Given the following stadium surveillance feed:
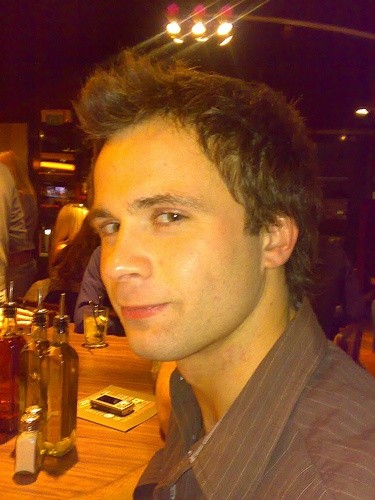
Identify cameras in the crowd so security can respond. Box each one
[91,394,135,416]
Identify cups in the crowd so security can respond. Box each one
[83,306,108,346]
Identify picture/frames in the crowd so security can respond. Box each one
[38,107,73,148]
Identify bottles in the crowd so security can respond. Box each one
[40,314,79,458]
[14,406,45,478]
[0,301,27,434]
[17,307,53,420]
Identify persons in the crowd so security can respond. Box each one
[41,210,101,321]
[0,162,37,308]
[74,245,125,336]
[41,203,89,312]
[70,48,375,499]
[0,150,37,284]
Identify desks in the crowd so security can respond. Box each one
[0,323,166,500]
